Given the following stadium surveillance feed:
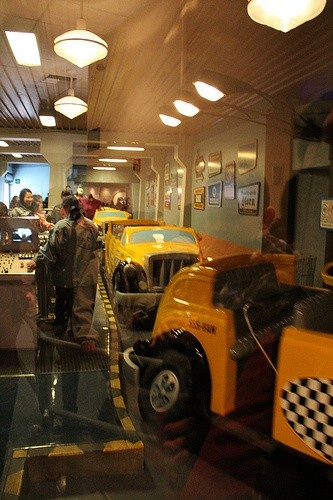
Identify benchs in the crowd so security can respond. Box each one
[210,262,333,335]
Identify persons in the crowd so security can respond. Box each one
[260,207,301,257]
[0,185,127,231]
[26,195,99,353]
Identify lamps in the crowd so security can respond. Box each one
[158,70,325,142]
[54,70,89,119]
[245,0,326,34]
[54,0,109,69]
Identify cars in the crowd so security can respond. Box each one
[92,207,332,476]
